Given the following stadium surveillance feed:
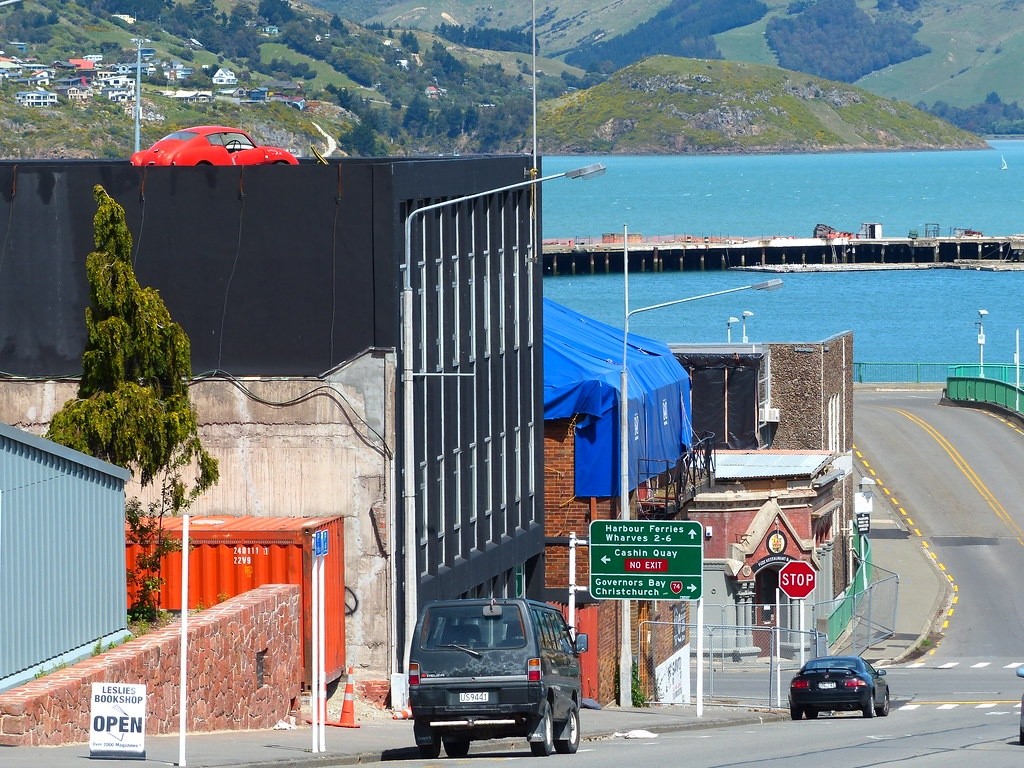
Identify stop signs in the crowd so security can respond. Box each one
[779,560,817,599]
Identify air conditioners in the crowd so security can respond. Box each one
[765,409,781,424]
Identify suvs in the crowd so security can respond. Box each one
[407,598,590,761]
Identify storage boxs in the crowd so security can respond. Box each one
[126,515,347,685]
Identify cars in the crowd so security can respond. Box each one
[789,654,890,720]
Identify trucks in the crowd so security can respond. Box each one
[121,516,346,704]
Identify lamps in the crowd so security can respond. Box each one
[727,317,739,341]
[742,311,753,343]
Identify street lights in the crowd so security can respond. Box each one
[403,163,608,677]
[129,37,152,152]
[619,222,783,706]
[977,310,990,378]
[727,315,740,342]
[741,311,754,342]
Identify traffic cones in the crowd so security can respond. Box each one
[304,672,337,726]
[393,705,414,720]
[331,668,363,729]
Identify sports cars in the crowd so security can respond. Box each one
[131,126,300,167]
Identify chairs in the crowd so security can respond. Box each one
[497,621,526,647]
[446,626,485,647]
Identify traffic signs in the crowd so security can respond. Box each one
[587,518,704,602]
